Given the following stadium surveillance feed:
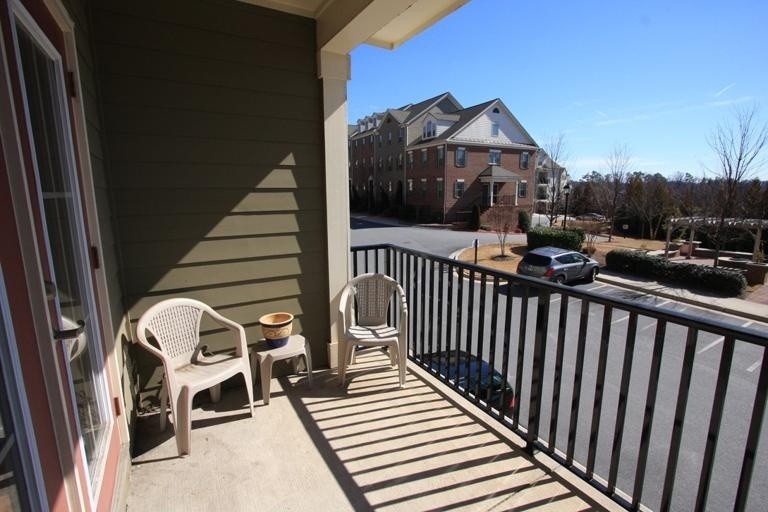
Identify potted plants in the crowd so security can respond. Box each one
[746,240,768,286]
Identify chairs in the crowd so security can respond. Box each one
[60,316,88,362]
[337,273,408,389]
[136,298,254,458]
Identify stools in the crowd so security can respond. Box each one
[250,334,312,405]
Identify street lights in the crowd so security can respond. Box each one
[564,183,570,230]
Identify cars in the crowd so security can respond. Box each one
[576,213,605,222]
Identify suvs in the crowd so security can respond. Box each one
[413,350,513,419]
[517,247,599,293]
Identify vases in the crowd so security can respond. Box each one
[258,312,294,347]
